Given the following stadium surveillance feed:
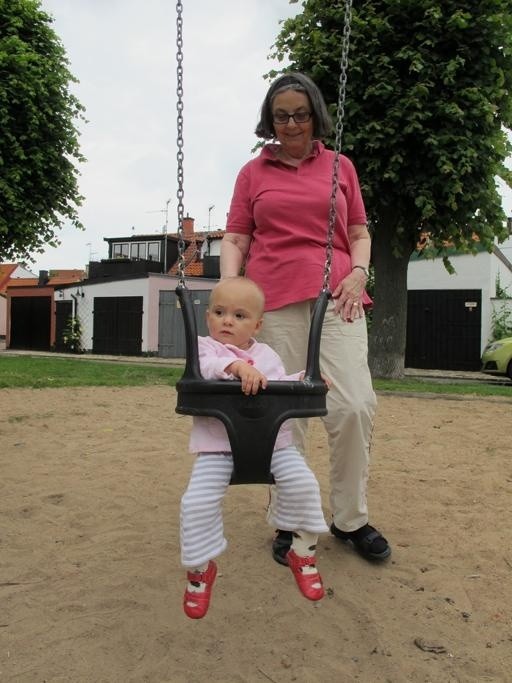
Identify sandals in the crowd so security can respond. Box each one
[329,520,392,560]
[273,528,293,566]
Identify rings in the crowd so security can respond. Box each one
[353,301,358,306]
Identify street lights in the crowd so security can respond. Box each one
[166,199,173,234]
[208,205,217,231]
[85,243,92,261]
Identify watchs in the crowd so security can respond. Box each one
[350,265,370,278]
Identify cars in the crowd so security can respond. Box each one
[481,336,511,380]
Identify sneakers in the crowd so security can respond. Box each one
[184,559,217,619]
[287,550,325,600]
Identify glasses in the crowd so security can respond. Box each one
[271,112,311,123]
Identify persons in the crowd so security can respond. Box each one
[220,72,389,570]
[177,277,328,620]
